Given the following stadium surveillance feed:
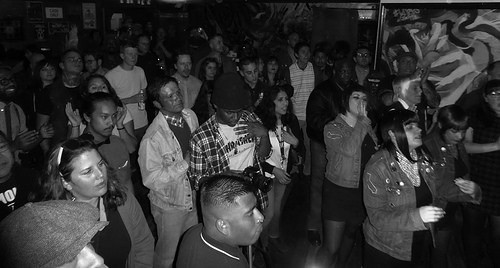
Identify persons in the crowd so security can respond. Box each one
[0,8,500,268]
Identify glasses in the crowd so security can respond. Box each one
[159,88,181,103]
[0,77,16,86]
[356,52,369,57]
[85,59,97,65]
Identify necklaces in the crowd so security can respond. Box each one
[216,122,239,156]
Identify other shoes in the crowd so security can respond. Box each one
[308,230,321,247]
[248,250,266,268]
[269,239,293,254]
[263,249,273,266]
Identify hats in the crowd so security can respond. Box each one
[264,84,294,98]
[211,73,252,111]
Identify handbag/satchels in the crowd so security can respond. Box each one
[18,166,39,197]
[289,130,307,178]
